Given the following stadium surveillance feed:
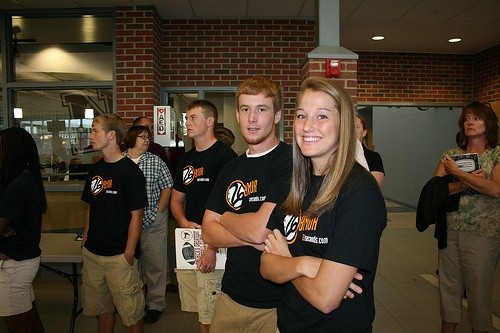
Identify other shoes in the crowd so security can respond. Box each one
[165,283,179,293]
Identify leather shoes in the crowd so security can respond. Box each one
[144,305,161,324]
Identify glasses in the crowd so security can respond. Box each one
[137,135,150,140]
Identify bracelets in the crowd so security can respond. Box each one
[459,182,463,189]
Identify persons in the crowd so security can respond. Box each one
[435,101,500,333]
[200,76,293,333]
[259,76,387,333]
[0,126,49,333]
[80,100,237,333]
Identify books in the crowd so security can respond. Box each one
[449,152,479,195]
[174,226,227,270]
